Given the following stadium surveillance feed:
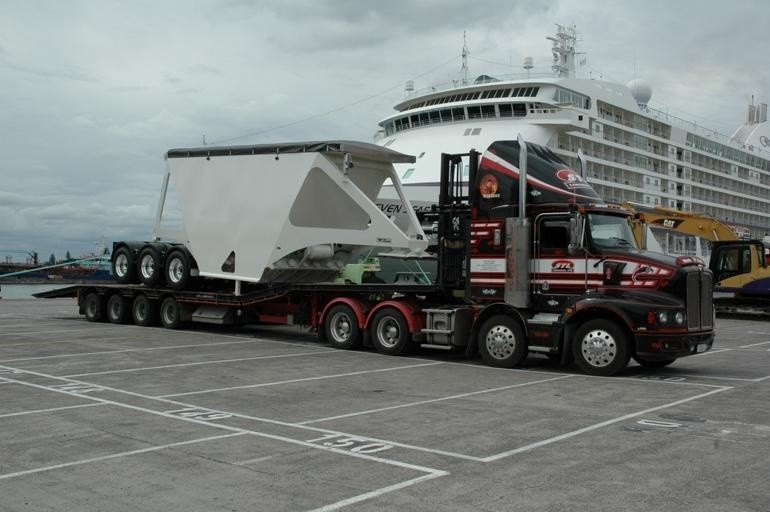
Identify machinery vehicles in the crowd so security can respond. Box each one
[614,201,769,312]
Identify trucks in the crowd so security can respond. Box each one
[33,136,714,377]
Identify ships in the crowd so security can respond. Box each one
[376,22,769,233]
